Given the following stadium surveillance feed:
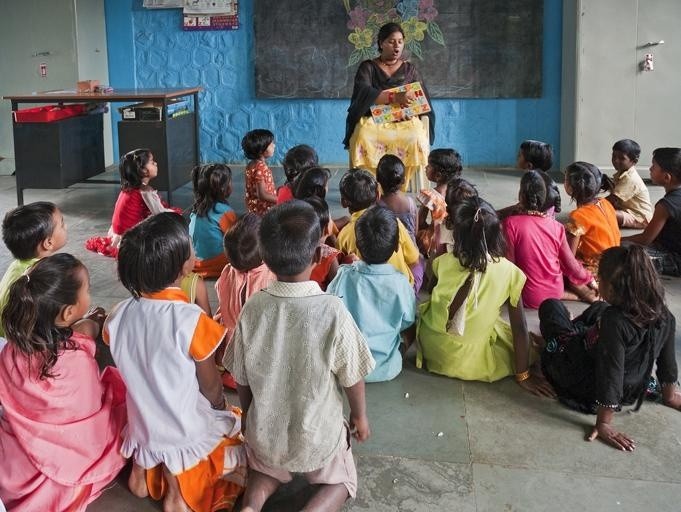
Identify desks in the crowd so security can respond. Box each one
[2,86,203,207]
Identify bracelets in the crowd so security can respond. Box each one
[513,370,531,385]
[388,92,394,104]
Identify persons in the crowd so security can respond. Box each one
[497,140,562,216]
[423,149,462,256]
[103,213,248,510]
[563,161,621,285]
[210,212,274,388]
[302,198,343,290]
[336,166,423,294]
[275,145,350,229]
[292,168,339,247]
[603,138,653,229]
[1,200,107,338]
[85,147,182,256]
[376,155,418,251]
[326,206,419,384]
[242,128,281,214]
[221,200,375,512]
[499,170,597,307]
[187,163,241,277]
[424,180,478,290]
[178,273,210,315]
[415,196,558,399]
[621,148,680,277]
[530,243,680,452]
[343,22,434,192]
[3,255,125,511]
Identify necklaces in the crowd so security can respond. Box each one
[377,55,401,67]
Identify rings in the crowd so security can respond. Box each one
[407,100,410,102]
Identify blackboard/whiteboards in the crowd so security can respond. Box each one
[251,0,544,101]
